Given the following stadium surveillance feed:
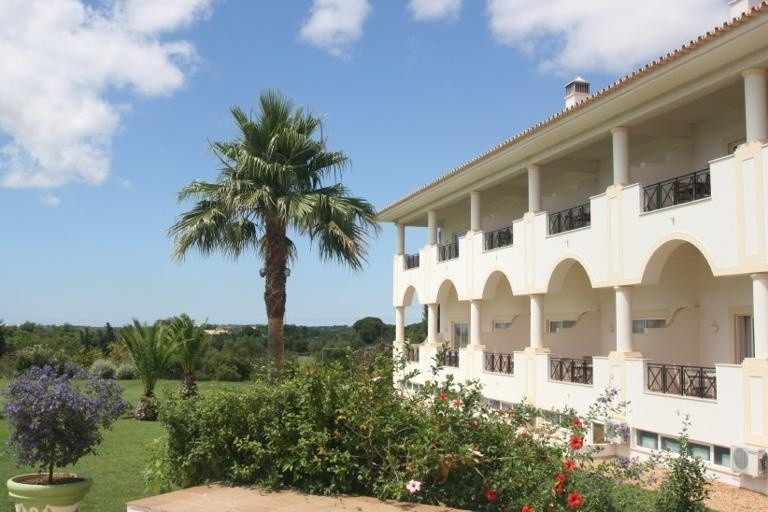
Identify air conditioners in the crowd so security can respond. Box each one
[730,444,767,478]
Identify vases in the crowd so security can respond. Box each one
[6,473,92,512]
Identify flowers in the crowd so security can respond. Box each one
[0,358,127,485]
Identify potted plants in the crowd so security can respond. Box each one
[121,318,195,420]
[162,313,217,401]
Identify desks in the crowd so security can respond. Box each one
[690,372,716,400]
[567,364,592,385]
[675,182,704,204]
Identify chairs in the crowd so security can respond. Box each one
[674,362,696,395]
